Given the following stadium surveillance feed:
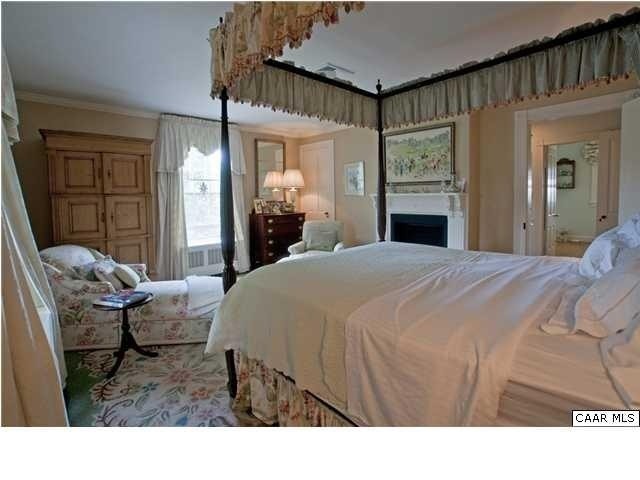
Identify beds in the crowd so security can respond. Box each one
[205,239,640,427]
[39,245,225,351]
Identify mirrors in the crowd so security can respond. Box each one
[254,138,286,204]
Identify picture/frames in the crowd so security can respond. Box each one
[556,157,575,188]
[381,122,455,185]
[253,200,264,214]
[344,161,365,197]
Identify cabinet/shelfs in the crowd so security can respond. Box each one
[38,129,157,281]
[249,212,306,271]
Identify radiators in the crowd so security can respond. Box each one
[181,241,238,276]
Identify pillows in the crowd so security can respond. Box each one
[305,230,337,251]
[579,213,640,280]
[539,245,640,338]
[93,261,125,291]
[114,263,140,288]
[72,253,116,282]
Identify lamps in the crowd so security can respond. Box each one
[263,171,284,201]
[280,169,305,213]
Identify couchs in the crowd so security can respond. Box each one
[288,220,345,260]
